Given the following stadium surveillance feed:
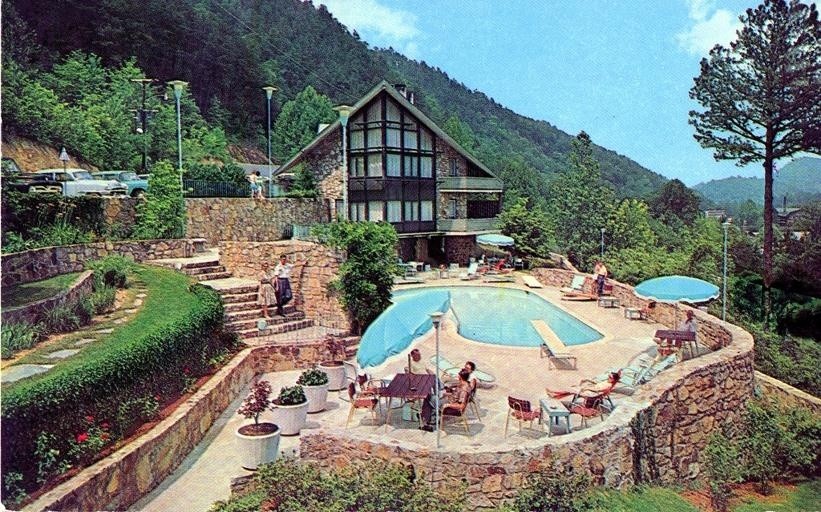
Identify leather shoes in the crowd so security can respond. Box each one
[416,412,424,420]
[420,426,431,431]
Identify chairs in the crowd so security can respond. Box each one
[624,301,658,325]
[346,373,388,427]
[559,273,587,293]
[560,368,622,431]
[439,377,482,432]
[578,343,689,396]
[504,396,544,441]
[390,255,543,289]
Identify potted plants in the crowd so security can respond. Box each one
[270,384,309,436]
[315,332,348,391]
[235,376,281,470]
[294,368,330,413]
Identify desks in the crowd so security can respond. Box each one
[538,398,571,438]
[597,296,621,308]
[381,373,436,437]
[655,329,699,358]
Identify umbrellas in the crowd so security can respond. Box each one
[633,276,721,329]
[477,234,514,263]
[356,290,453,369]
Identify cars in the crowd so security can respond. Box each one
[1,156,154,200]
[130,173,154,180]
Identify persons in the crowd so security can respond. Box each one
[544,373,618,406]
[481,251,512,271]
[255,171,265,199]
[417,369,470,432]
[274,255,308,315]
[675,310,697,349]
[592,259,608,295]
[405,348,443,394]
[250,171,258,198]
[465,361,477,399]
[259,262,277,319]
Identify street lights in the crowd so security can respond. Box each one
[165,78,190,193]
[329,101,355,222]
[719,222,732,321]
[260,86,278,197]
[599,228,606,256]
[129,76,168,174]
[426,310,447,447]
